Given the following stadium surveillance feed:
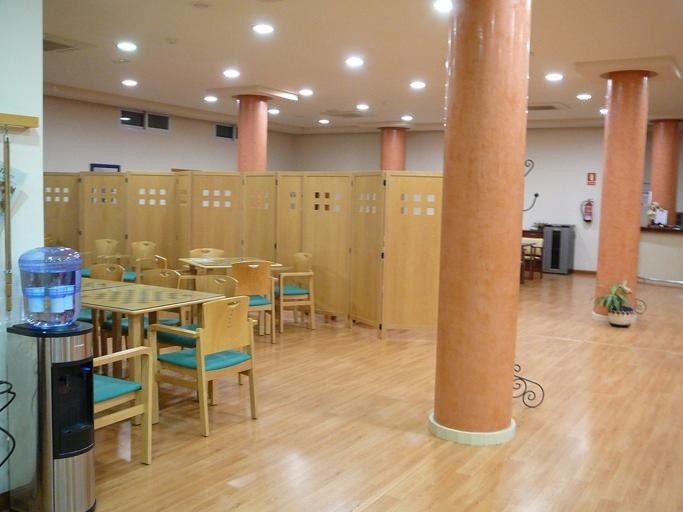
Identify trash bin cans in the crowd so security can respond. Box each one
[544,225,575,274]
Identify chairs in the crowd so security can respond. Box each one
[80,267,239,425]
[79,238,316,346]
[520,230,544,282]
[148,295,260,437]
[79,343,154,465]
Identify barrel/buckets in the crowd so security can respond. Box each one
[19,245,83,333]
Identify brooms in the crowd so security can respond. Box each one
[1,112,40,313]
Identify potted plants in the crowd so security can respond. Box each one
[592,281,636,327]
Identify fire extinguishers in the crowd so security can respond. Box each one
[581,199,594,221]
